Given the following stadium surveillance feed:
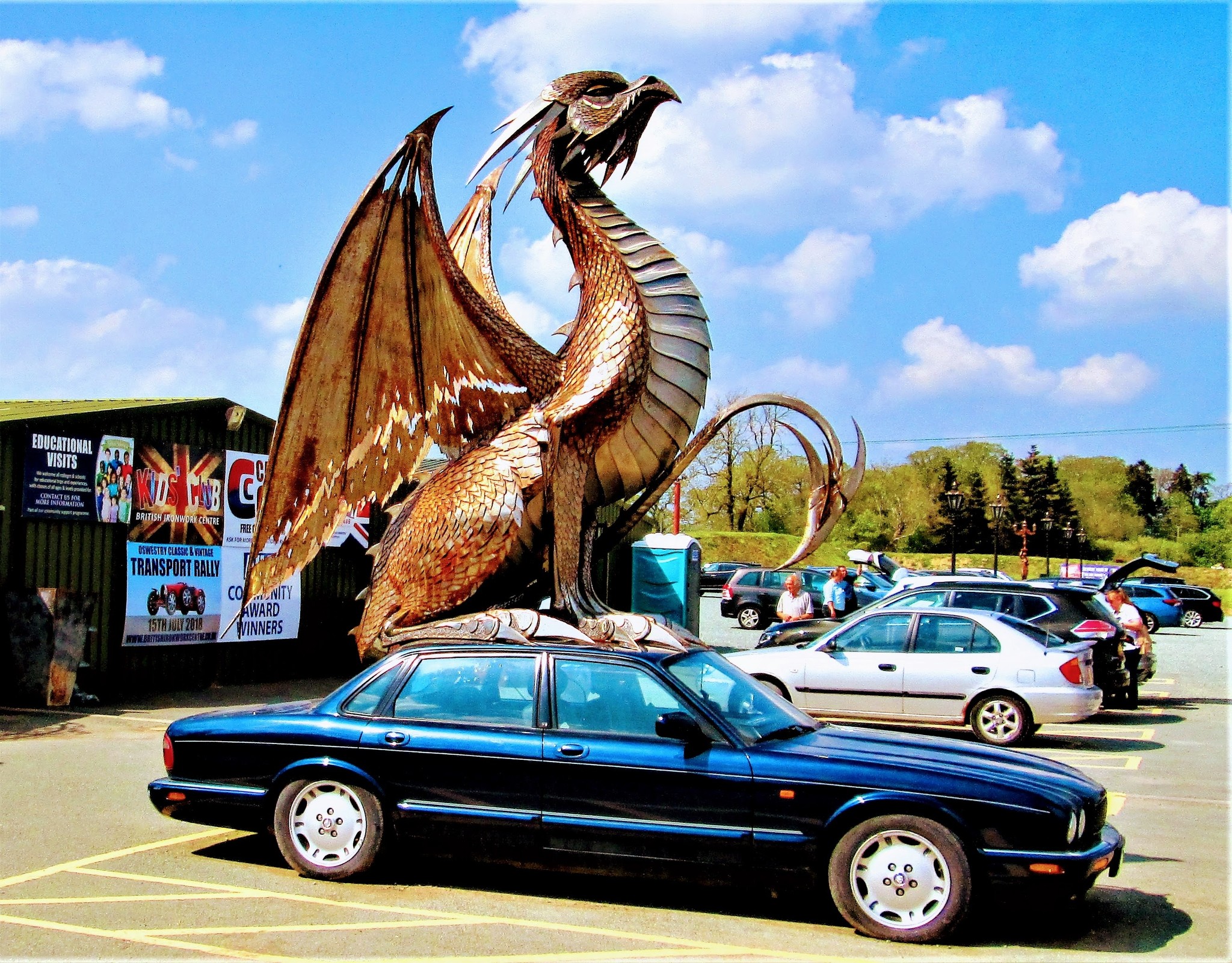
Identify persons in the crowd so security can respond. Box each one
[95,449,132,523]
[1107,590,1152,710]
[822,564,862,618]
[776,574,814,622]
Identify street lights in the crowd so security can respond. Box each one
[1039,511,1055,578]
[1062,522,1074,577]
[988,493,1008,576]
[1013,519,1037,580]
[944,480,964,574]
[1076,527,1088,577]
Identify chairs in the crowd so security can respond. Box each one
[524,668,571,727]
[593,668,633,732]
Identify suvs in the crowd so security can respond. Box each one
[699,562,762,597]
[1023,551,1224,635]
[752,550,1180,705]
[799,548,1014,607]
[721,567,833,631]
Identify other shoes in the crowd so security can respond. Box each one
[1125,693,1138,710]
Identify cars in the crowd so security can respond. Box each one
[146,582,205,616]
[698,607,1104,747]
[148,642,1126,946]
